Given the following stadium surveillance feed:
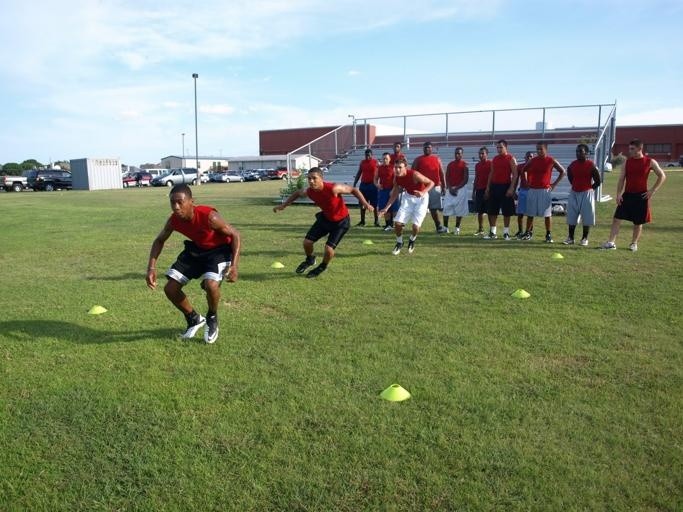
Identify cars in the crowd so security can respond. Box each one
[121,167,302,187]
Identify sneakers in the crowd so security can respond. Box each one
[182,314,206,338]
[374,221,381,227]
[382,224,394,231]
[562,236,574,245]
[391,241,404,256]
[474,231,485,236]
[512,231,533,240]
[356,222,366,226]
[503,232,511,240]
[205,315,219,343]
[306,263,327,278]
[454,227,460,236]
[296,256,316,273]
[601,241,616,250]
[580,237,589,245]
[629,243,638,251]
[436,224,449,233]
[545,233,554,243]
[483,229,498,239]
[407,238,415,253]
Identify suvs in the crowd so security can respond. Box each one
[27,170,72,191]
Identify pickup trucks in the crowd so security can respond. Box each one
[0,170,32,193]
[665,156,682,167]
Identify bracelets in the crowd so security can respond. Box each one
[147,267,156,270]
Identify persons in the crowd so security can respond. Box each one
[597,140,666,252]
[562,144,601,245]
[352,140,566,255]
[273,167,375,278]
[135,172,142,188]
[146,183,241,344]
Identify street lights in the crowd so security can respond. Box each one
[191,72,201,175]
[181,133,186,159]
